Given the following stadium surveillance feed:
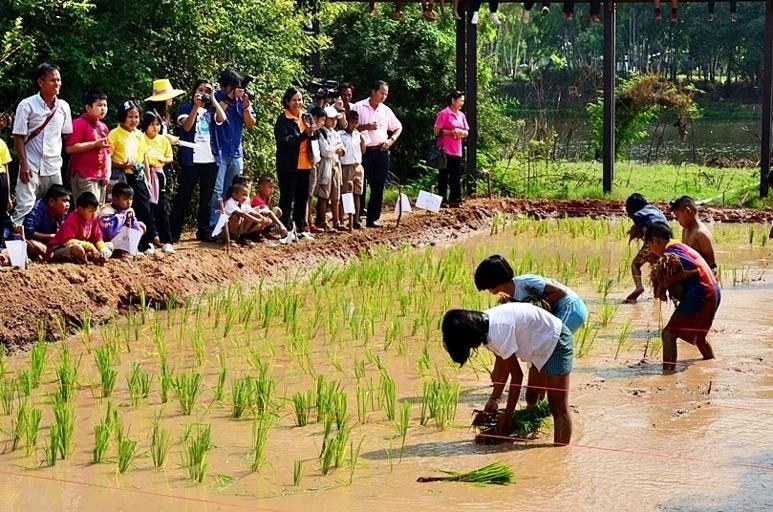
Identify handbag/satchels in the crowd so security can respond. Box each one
[425,144,448,169]
[307,130,322,165]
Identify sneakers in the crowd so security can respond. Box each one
[137,242,156,256]
[304,224,325,234]
[160,243,176,254]
[230,230,315,250]
[322,225,338,234]
[333,225,350,231]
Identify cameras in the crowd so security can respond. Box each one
[134,163,143,171]
[198,94,211,104]
[241,74,256,100]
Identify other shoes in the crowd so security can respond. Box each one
[395,8,404,17]
[452,11,461,20]
[590,10,600,23]
[565,12,573,21]
[424,10,435,20]
[654,12,661,20]
[707,15,713,22]
[489,13,501,25]
[369,7,375,16]
[523,10,530,24]
[541,7,549,16]
[471,14,479,24]
[728,16,736,21]
[441,7,448,18]
[670,14,678,21]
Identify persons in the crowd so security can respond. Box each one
[442,301,576,449]
[274,80,403,245]
[645,222,721,374]
[669,196,719,279]
[212,173,288,250]
[473,255,587,413]
[620,193,669,304]
[432,89,470,209]
[1,61,256,265]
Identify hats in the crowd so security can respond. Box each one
[323,106,342,119]
[143,78,187,103]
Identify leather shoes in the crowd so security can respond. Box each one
[448,200,464,208]
[366,219,383,228]
[346,216,363,227]
[440,202,450,208]
